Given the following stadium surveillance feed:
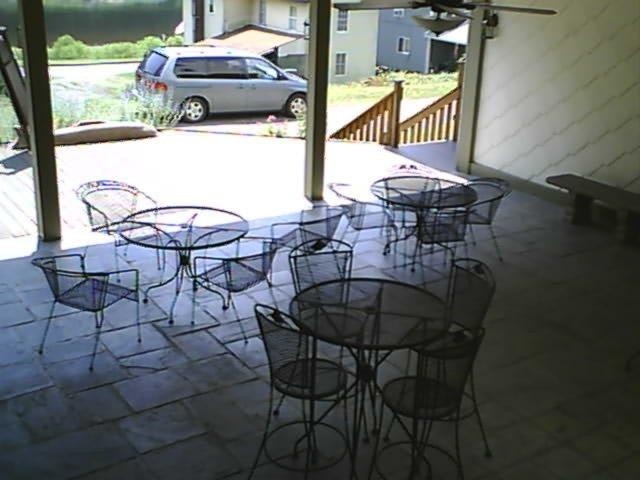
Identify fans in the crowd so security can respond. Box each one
[333,1,557,23]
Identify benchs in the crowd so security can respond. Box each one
[545,173,640,245]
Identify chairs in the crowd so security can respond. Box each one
[31,171,512,480]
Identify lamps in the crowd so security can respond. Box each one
[409,7,466,38]
[483,12,500,39]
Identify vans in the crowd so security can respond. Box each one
[132,41,319,135]
